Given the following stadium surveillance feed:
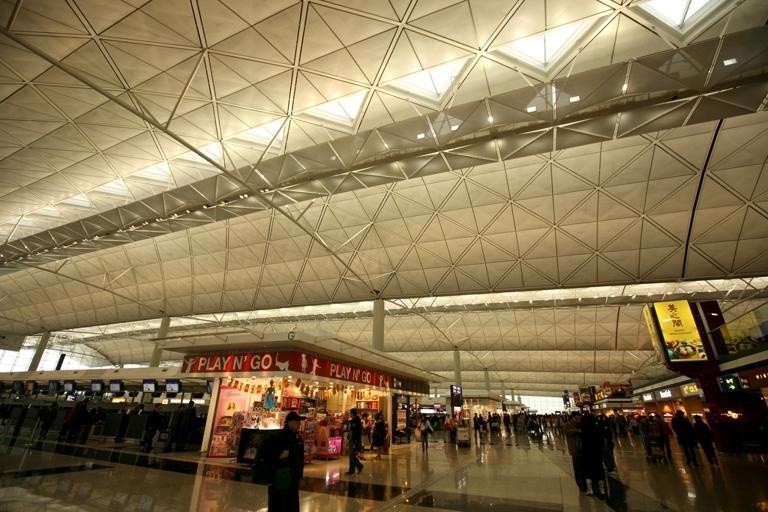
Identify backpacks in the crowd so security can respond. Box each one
[249,432,291,486]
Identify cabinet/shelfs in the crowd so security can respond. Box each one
[357,400,383,421]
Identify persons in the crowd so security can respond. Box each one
[28,398,196,454]
[472,409,719,466]
[263,411,306,512]
[566,412,608,499]
[343,408,386,474]
[417,414,440,451]
[263,379,275,411]
[445,414,457,444]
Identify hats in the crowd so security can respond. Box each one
[284,411,308,422]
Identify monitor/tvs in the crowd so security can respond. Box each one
[48,380,61,394]
[109,379,124,393]
[143,379,158,393]
[25,380,37,391]
[165,379,182,393]
[64,380,76,392]
[91,379,105,392]
[207,381,213,394]
